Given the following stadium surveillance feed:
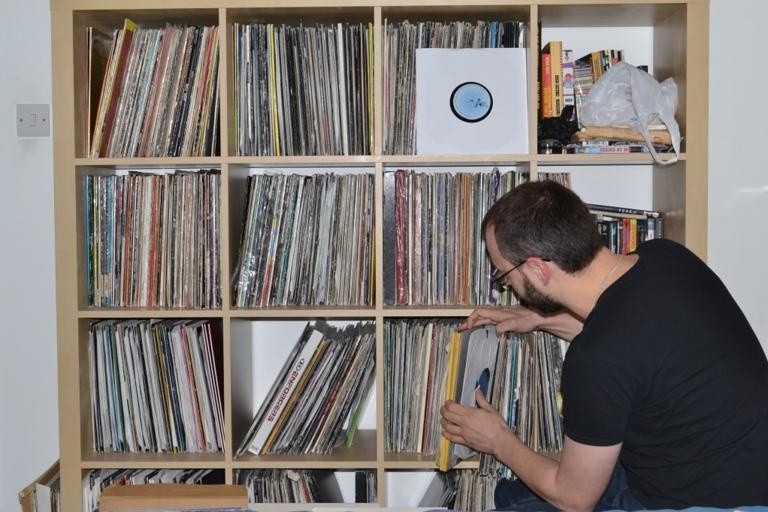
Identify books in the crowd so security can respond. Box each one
[537,172,571,191]
[233,319,375,458]
[440,468,524,512]
[81,468,225,511]
[75,15,222,158]
[383,169,533,307]
[235,172,373,310]
[583,200,666,256]
[16,461,58,511]
[86,323,223,453]
[232,20,372,157]
[385,15,521,155]
[383,315,567,483]
[237,468,376,503]
[84,171,220,311]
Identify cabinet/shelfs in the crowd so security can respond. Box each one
[50,1,707,512]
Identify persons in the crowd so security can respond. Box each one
[538,22,681,153]
[441,182,768,512]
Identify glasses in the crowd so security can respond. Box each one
[486,253,557,294]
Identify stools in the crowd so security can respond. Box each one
[101,484,249,512]
